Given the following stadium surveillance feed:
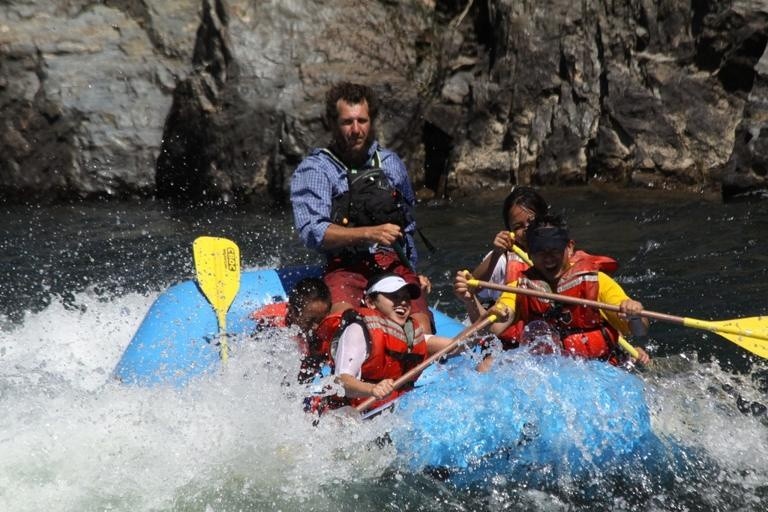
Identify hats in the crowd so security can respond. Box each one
[365,272,421,300]
[524,226,569,254]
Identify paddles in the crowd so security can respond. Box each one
[467,279,767,359]
[191,236,240,362]
[319,303,505,428]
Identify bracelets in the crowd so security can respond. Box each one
[630,318,640,320]
[464,300,474,305]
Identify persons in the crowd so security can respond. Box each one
[290,80,437,336]
[453,216,649,369]
[307,270,509,427]
[472,186,650,376]
[249,277,344,386]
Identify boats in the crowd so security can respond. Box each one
[110,267,697,496]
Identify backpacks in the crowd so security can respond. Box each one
[320,146,437,273]
